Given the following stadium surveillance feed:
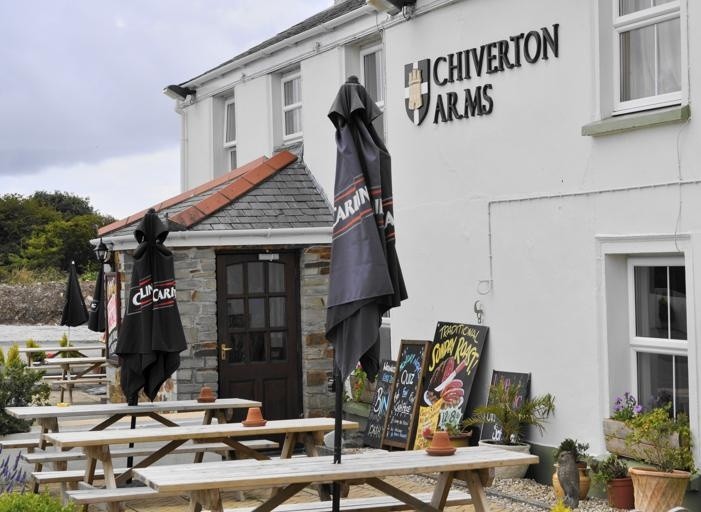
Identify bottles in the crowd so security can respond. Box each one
[431,432,449,449]
[246,408,263,421]
[201,388,212,397]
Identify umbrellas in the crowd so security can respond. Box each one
[115,208,187,481]
[88,259,115,332]
[324,76,410,512]
[59,260,90,403]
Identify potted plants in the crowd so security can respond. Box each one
[459,376,556,480]
[553,391,700,512]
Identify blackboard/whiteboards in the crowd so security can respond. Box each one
[103,272,122,367]
[380,340,433,449]
[364,358,398,449]
[480,369,531,441]
[411,321,490,450]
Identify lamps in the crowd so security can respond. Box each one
[163,83,197,102]
[92,235,111,262]
[366,0,419,18]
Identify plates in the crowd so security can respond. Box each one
[199,397,216,402]
[242,421,266,426]
[426,448,455,455]
